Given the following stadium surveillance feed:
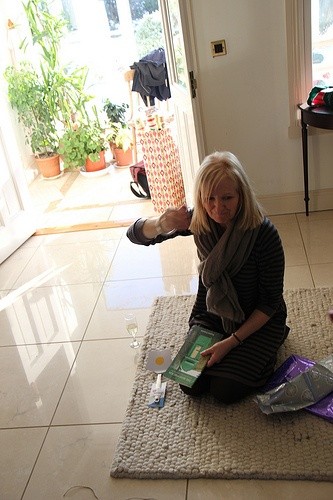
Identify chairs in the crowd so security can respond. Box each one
[124,49,169,162]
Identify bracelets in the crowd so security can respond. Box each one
[232,333,243,346]
[154,213,177,236]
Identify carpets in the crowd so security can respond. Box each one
[110,287,333,482]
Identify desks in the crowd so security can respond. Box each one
[297,103,333,217]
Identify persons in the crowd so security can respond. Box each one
[126,152,290,408]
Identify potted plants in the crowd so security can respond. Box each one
[3,0,134,178]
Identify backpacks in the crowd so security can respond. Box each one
[129,159,151,199]
